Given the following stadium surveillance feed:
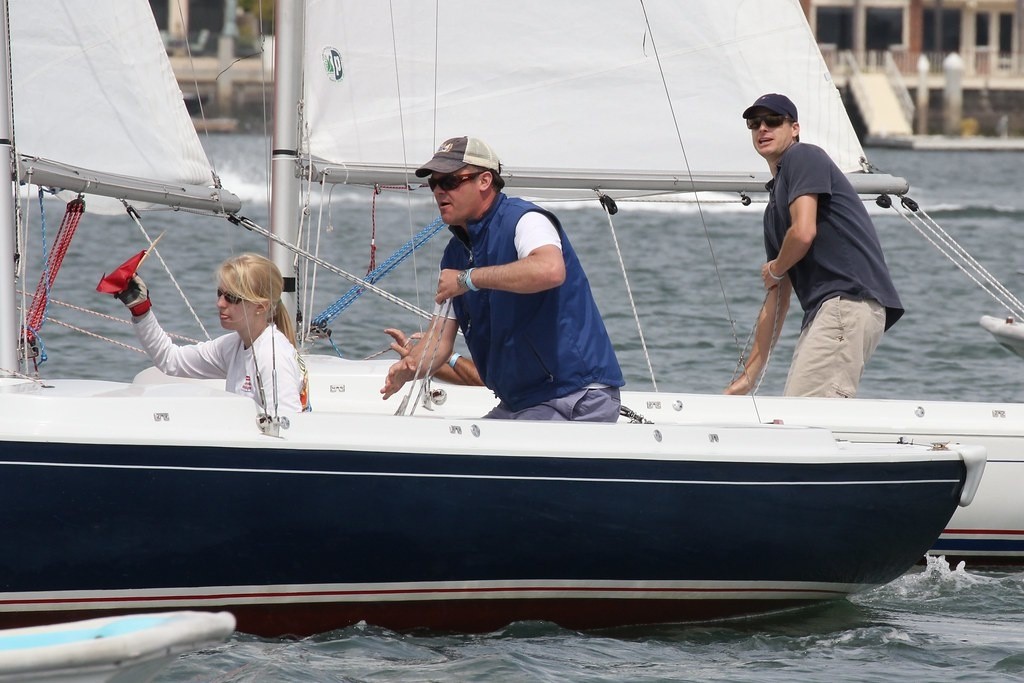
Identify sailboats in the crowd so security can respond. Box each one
[130,0,1024,566]
[0,0,992,638]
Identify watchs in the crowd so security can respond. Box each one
[456,270,467,287]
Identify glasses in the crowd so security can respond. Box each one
[747,114,785,129]
[428,172,484,191]
[217,287,242,303]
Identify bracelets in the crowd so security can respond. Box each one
[466,268,480,292]
[768,259,785,279]
[129,299,152,316]
[449,352,462,367]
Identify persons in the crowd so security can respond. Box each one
[724,93,905,398]
[379,138,625,423]
[383,328,485,386]
[113,252,304,414]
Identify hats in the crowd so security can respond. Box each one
[415,136,500,178]
[742,94,798,123]
